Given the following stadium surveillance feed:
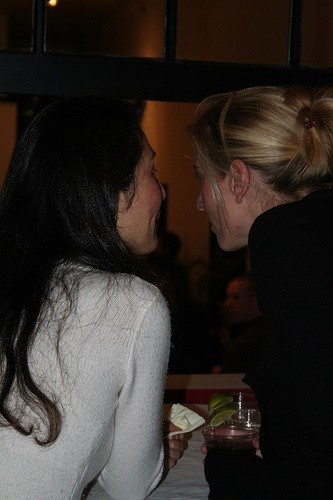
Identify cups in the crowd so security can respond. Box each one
[201,427,257,450]
[225,393,261,434]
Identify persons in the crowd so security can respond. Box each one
[187,86,332,499]
[0,103,191,499]
